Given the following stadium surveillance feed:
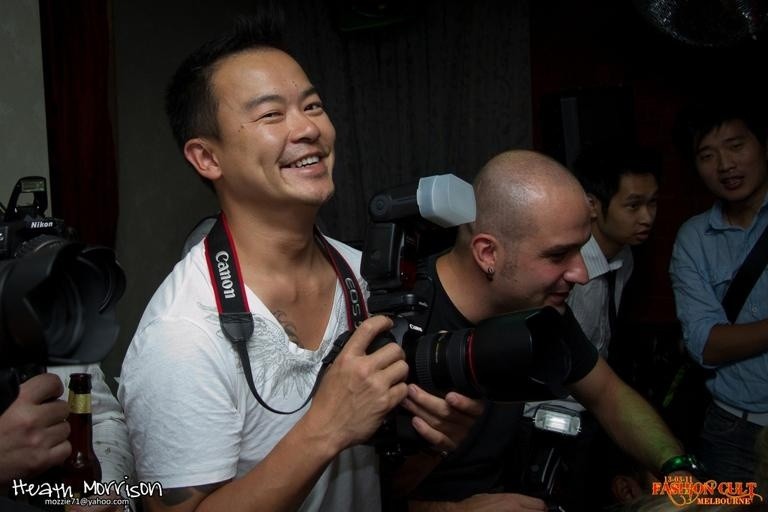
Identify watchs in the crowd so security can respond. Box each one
[656,452,709,483]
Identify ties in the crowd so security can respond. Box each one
[605,272,618,339]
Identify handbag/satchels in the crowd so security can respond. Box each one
[658,382,712,471]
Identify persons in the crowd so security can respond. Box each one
[113,32,488,511]
[375,147,711,512]
[606,427,696,504]
[2,361,140,510]
[665,95,767,512]
[561,147,665,377]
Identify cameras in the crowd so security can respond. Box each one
[0,175,127,414]
[324,172,598,462]
